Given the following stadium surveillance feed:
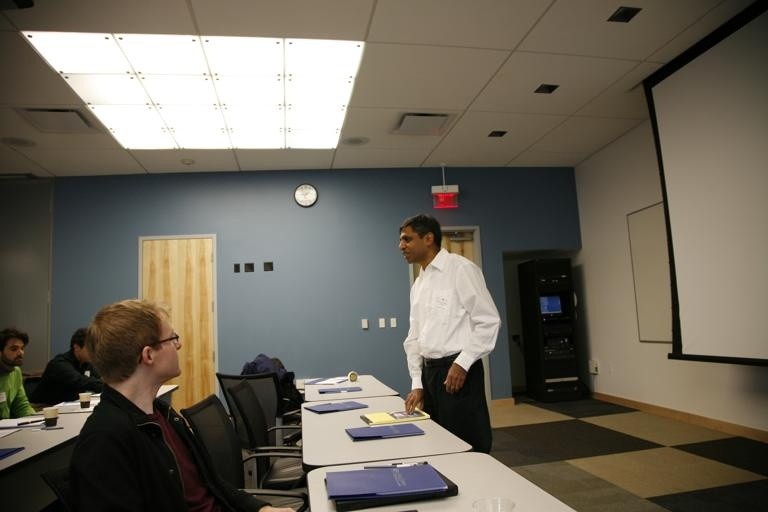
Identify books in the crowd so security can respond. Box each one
[359,407,431,426]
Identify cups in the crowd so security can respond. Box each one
[79,393,91,408]
[43,407,58,426]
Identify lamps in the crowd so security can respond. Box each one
[20,29,365,150]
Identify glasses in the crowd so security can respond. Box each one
[139,333,179,365]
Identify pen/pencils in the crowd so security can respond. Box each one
[18,419,46,425]
[365,465,411,469]
[41,427,63,430]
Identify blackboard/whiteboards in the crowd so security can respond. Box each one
[626,201,673,343]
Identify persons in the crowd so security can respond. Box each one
[41,327,103,405]
[66,300,292,512]
[399,213,502,455]
[0,330,37,418]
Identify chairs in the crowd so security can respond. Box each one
[180,394,308,512]
[218,372,302,476]
[23,376,40,399]
[42,469,72,512]
[228,378,305,489]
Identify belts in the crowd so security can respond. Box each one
[424,357,456,367]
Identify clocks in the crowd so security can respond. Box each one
[294,183,318,207]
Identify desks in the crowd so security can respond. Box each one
[307,452,575,512]
[52,397,100,413]
[304,375,399,402]
[91,384,179,405]
[0,412,92,471]
[301,395,472,473]
